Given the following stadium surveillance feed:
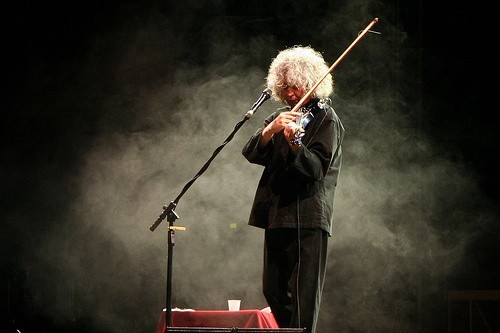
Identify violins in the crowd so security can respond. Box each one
[288,97,332,150]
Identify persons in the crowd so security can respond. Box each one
[241,45,346,333]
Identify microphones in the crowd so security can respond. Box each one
[246,88,273,118]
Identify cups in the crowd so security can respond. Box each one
[227,300,241,311]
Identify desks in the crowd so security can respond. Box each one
[156,309,280,333]
